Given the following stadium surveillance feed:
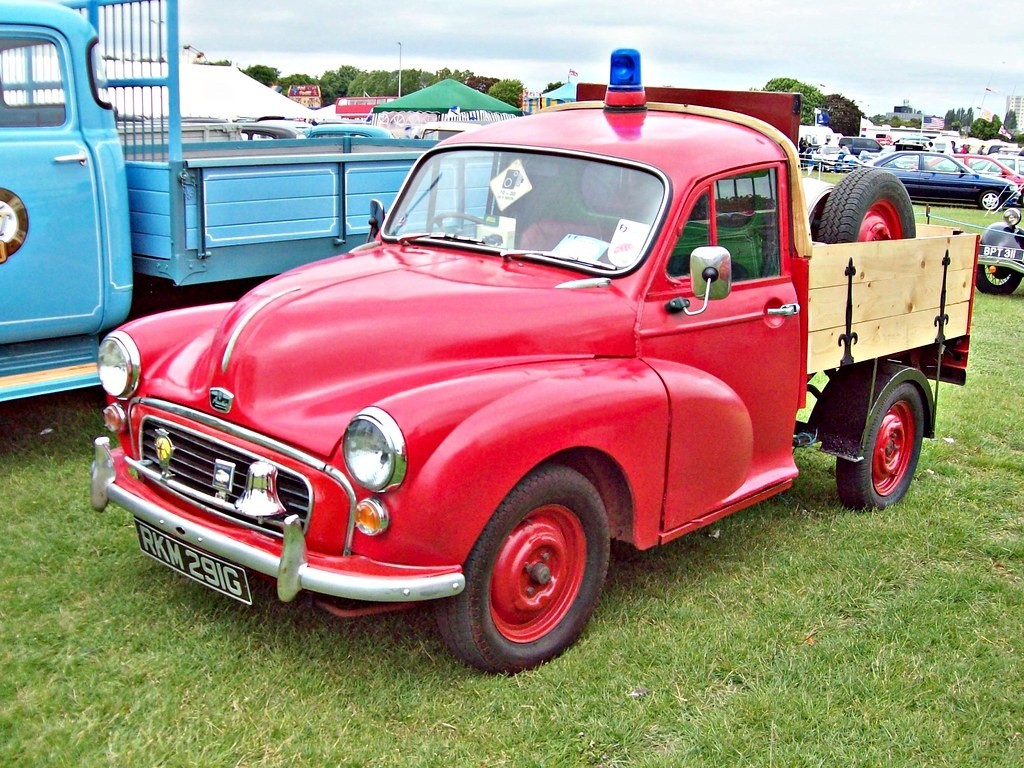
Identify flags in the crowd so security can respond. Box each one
[922,117,945,130]
[569,69,578,77]
[998,125,1012,140]
[860,118,874,136]
[817,113,829,123]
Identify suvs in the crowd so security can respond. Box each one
[839,137,888,159]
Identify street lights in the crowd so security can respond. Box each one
[398,41,402,100]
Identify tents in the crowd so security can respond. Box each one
[373,78,525,140]
[541,83,576,109]
[0,53,317,122]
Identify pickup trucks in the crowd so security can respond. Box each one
[91,56,981,676]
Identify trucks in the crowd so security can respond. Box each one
[0,0,496,404]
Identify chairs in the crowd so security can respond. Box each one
[516,218,613,254]
[723,212,776,283]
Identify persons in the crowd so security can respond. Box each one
[831,145,854,174]
[977,145,985,155]
[798,137,807,170]
[951,140,957,153]
[928,142,937,152]
[960,144,971,154]
[806,143,814,176]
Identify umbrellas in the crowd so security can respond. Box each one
[901,137,930,144]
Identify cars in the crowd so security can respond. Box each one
[810,146,855,172]
[914,155,1024,207]
[857,149,1021,210]
[878,136,1024,175]
[237,120,482,139]
[976,208,1024,295]
